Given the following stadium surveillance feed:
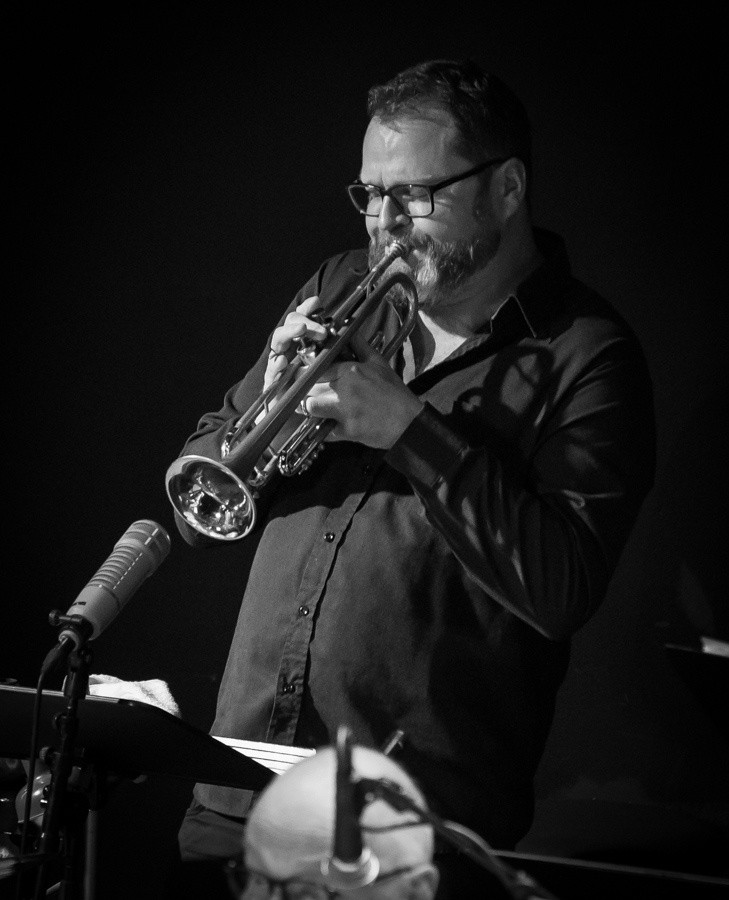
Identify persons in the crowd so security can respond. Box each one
[173,60,657,900]
[237,745,439,900]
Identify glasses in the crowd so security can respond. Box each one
[346,151,533,218]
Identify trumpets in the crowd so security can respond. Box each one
[162,237,421,543]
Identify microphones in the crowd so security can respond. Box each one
[320,733,381,890]
[40,518,172,672]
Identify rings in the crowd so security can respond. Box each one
[300,395,310,418]
